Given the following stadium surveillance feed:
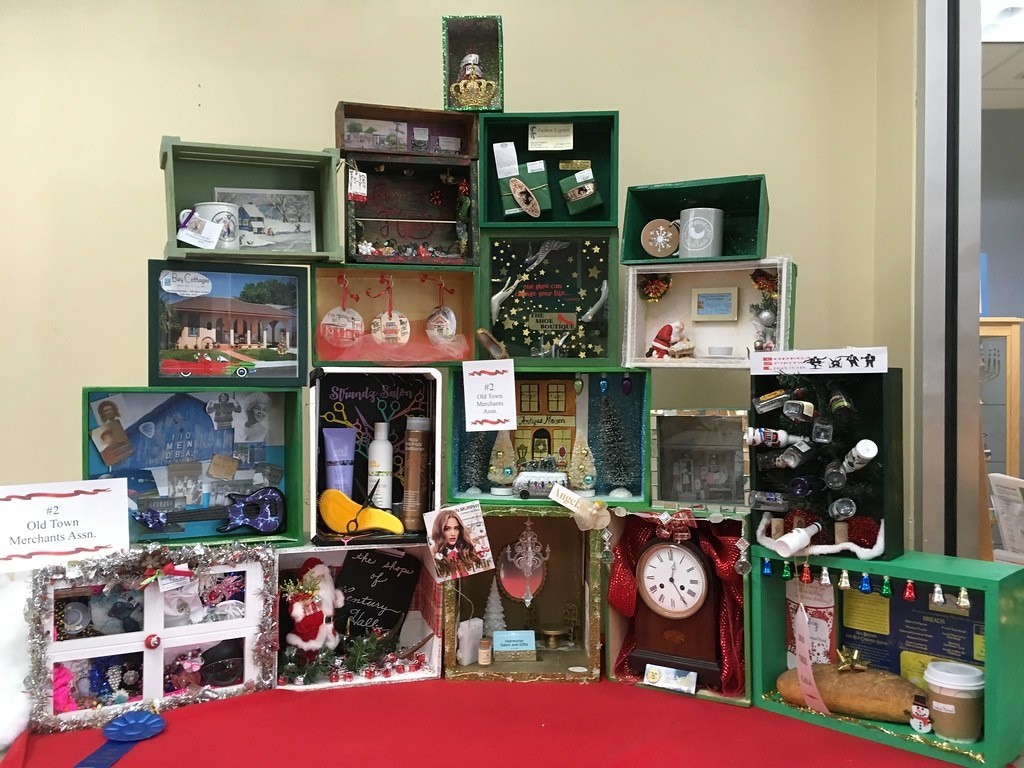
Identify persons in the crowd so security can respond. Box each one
[646,321,684,358]
[97,401,126,430]
[169,475,195,496]
[432,509,490,577]
[208,393,241,430]
[100,430,134,466]
[191,473,202,504]
[286,557,345,667]
[243,393,273,444]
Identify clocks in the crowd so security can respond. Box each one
[635,541,708,621]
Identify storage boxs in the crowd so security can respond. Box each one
[80,13,1024,767]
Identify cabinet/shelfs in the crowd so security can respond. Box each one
[979,317,1024,552]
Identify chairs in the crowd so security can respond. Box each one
[520,603,544,638]
[562,602,578,644]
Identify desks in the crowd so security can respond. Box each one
[540,623,569,648]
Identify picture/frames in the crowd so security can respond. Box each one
[146,257,310,390]
[690,286,738,321]
[34,560,263,716]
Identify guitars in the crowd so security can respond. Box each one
[130,486,286,533]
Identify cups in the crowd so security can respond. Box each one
[179,202,240,249]
[923,661,985,744]
[670,208,724,258]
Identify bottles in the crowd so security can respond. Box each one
[828,497,856,522]
[752,386,807,415]
[824,456,846,490]
[783,400,819,422]
[746,427,810,448]
[842,439,878,473]
[792,473,818,497]
[782,440,819,468]
[748,490,796,512]
[828,379,855,418]
[775,522,823,558]
[811,415,833,443]
